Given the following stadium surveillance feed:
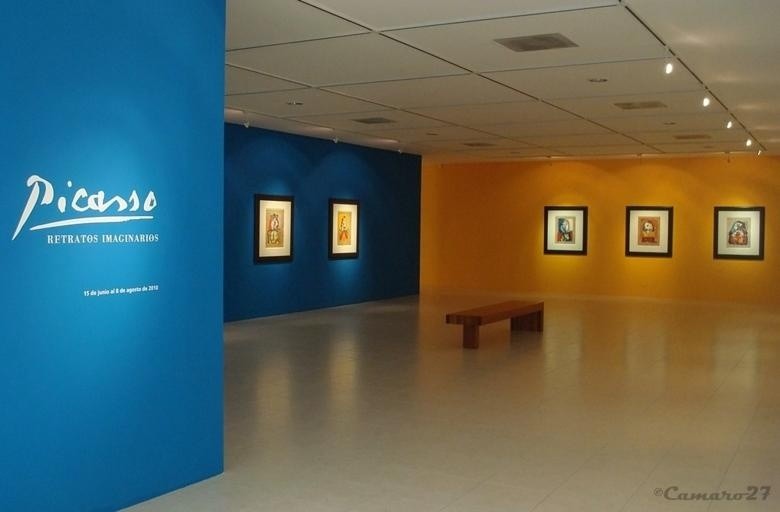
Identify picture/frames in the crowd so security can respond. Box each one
[254,193,361,265]
[542,204,588,256]
[624,206,674,258]
[712,204,766,261]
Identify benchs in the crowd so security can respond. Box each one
[445,300,543,351]
